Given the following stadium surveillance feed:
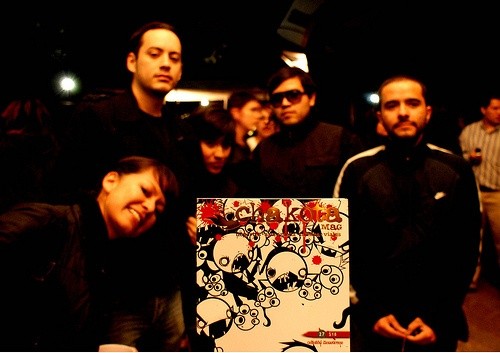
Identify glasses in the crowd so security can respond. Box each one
[269,89,309,107]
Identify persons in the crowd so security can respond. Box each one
[179,105,244,345]
[334,73,484,352]
[460,92,500,294]
[245,103,280,151]
[222,90,265,196]
[244,66,361,198]
[0,158,180,352]
[74,21,214,351]
